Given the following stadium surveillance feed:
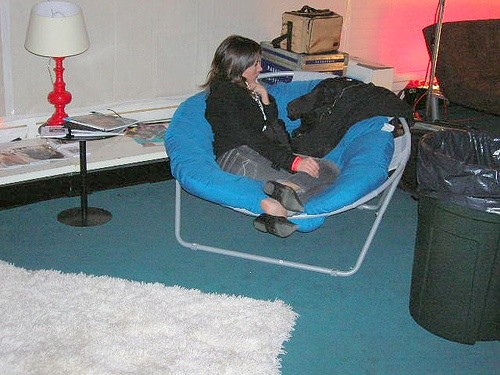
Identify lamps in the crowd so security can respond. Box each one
[24,1,90,134]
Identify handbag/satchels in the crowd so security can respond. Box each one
[271,4,343,54]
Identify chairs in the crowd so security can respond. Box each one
[164,70,411,277]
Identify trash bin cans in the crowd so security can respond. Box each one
[405,131,499,348]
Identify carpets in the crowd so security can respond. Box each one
[1,259,301,374]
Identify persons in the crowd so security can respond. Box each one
[198,35,339,239]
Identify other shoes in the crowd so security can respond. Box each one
[263,179,306,213]
[254,213,298,238]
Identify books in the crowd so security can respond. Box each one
[64,112,137,132]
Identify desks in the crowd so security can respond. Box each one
[38,120,118,227]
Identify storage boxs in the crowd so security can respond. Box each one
[259,42,349,85]
